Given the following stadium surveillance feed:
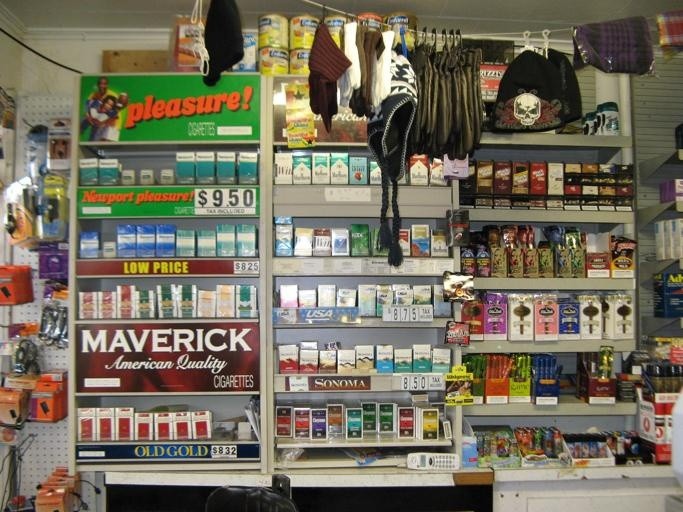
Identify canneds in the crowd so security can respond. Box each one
[258,12,416,75]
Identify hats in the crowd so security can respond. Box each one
[366,50,417,181]
[204,0,244,85]
[491,48,582,132]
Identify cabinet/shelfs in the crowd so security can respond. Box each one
[67,66,269,487]
[460,68,646,479]
[265,66,459,488]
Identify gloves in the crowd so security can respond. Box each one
[412,43,484,160]
[337,22,394,118]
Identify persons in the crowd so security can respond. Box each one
[80,77,129,141]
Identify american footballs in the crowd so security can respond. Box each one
[117,92,129,109]
